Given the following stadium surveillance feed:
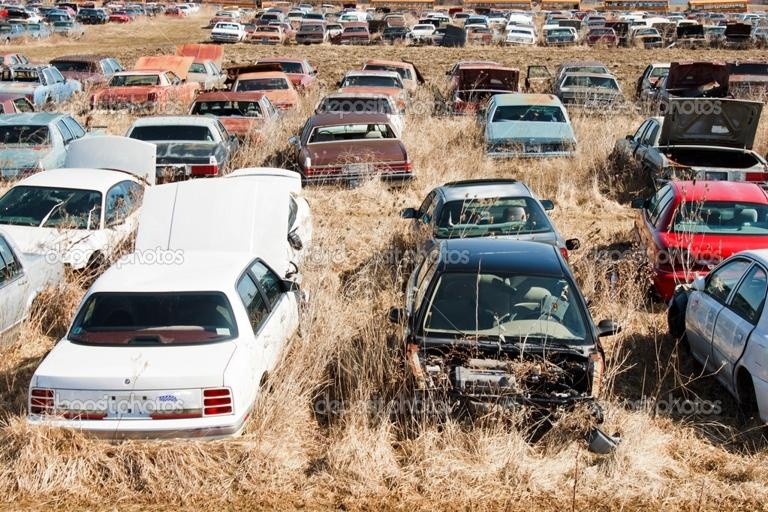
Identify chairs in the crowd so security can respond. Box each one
[432,278,482,332]
[511,286,549,320]
[461,272,511,316]
[502,207,525,222]
[738,207,759,223]
[363,130,384,139]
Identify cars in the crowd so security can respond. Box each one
[223,167,314,280]
[0,135,158,288]
[666,246,768,433]
[390,233,620,424]
[0,227,65,360]
[632,176,768,303]
[27,181,320,438]
[398,178,571,262]
